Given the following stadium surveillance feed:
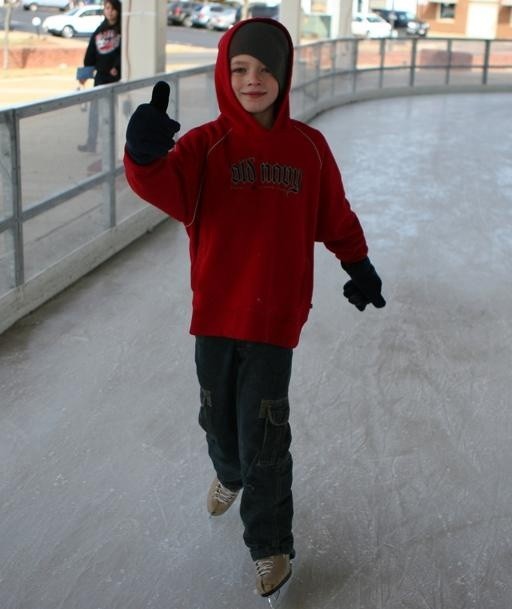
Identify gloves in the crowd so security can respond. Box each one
[342,257,386,311]
[125,81,182,166]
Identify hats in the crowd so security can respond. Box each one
[228,23,290,91]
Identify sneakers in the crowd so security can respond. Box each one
[206,476,239,516]
[77,141,97,153]
[255,552,292,597]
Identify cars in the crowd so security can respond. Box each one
[22,0,70,11]
[42,5,106,37]
[351,12,397,40]
[166,1,237,31]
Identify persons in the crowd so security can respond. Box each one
[125,15,386,598]
[76,1,122,154]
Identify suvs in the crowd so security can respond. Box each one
[372,8,429,40]
